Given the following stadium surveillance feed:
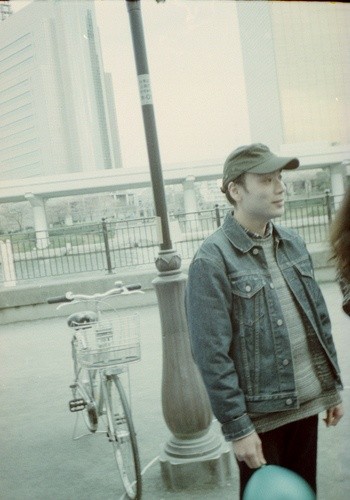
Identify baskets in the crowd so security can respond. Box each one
[72,311,141,369]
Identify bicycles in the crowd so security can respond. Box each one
[46,279,145,500]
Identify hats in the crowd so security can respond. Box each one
[221,142,300,193]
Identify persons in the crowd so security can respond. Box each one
[186,143,344,500]
